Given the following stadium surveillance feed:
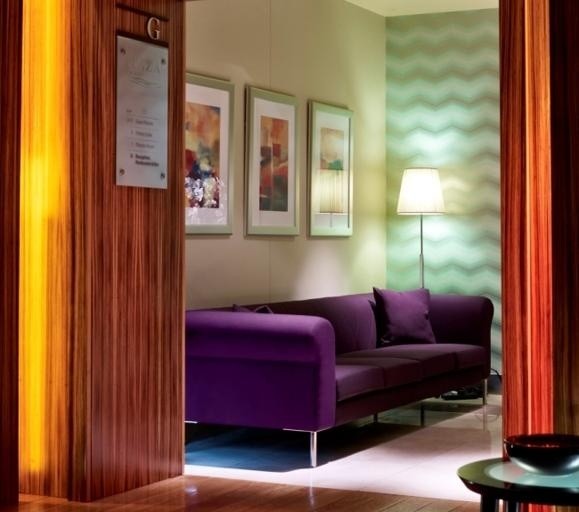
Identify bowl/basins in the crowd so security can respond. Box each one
[503,434,579,476]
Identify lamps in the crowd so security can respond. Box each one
[395,167,446,290]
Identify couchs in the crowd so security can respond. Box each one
[185,292,495,468]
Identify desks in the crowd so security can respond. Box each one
[458,456,579,512]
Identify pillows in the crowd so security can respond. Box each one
[301,294,380,355]
[371,287,438,347]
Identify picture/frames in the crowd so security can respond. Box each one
[307,99,355,238]
[243,85,302,237]
[184,71,234,236]
